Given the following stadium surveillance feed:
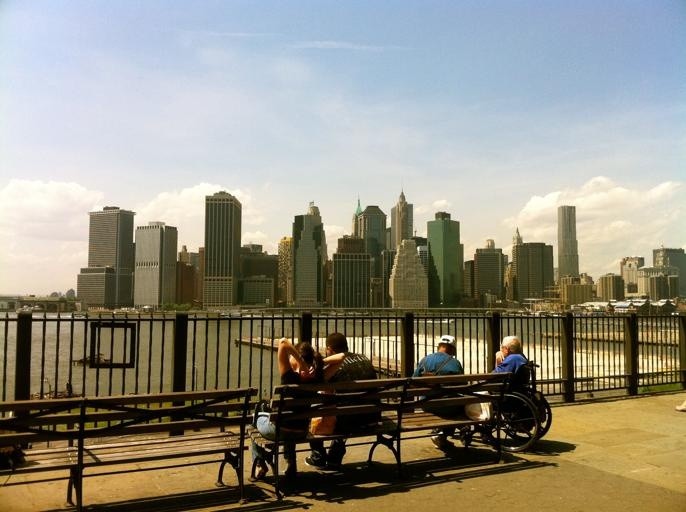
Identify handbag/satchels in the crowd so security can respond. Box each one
[310,414,337,437]
[465,391,494,421]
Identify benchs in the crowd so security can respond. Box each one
[248,371,516,498]
[0,384,258,512]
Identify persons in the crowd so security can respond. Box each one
[475,335,528,422]
[304,333,378,471]
[676,399,686,412]
[247,336,346,482]
[412,335,472,450]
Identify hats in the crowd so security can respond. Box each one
[438,335,456,350]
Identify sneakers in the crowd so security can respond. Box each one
[429,429,473,450]
[254,454,343,479]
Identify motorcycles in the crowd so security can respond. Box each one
[220,311,241,318]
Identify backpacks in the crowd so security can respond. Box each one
[420,355,453,387]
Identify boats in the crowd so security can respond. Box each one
[70,353,111,366]
[443,319,454,323]
[29,384,81,401]
[15,304,32,315]
[71,312,90,318]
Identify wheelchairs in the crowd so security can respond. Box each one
[463,360,552,453]
